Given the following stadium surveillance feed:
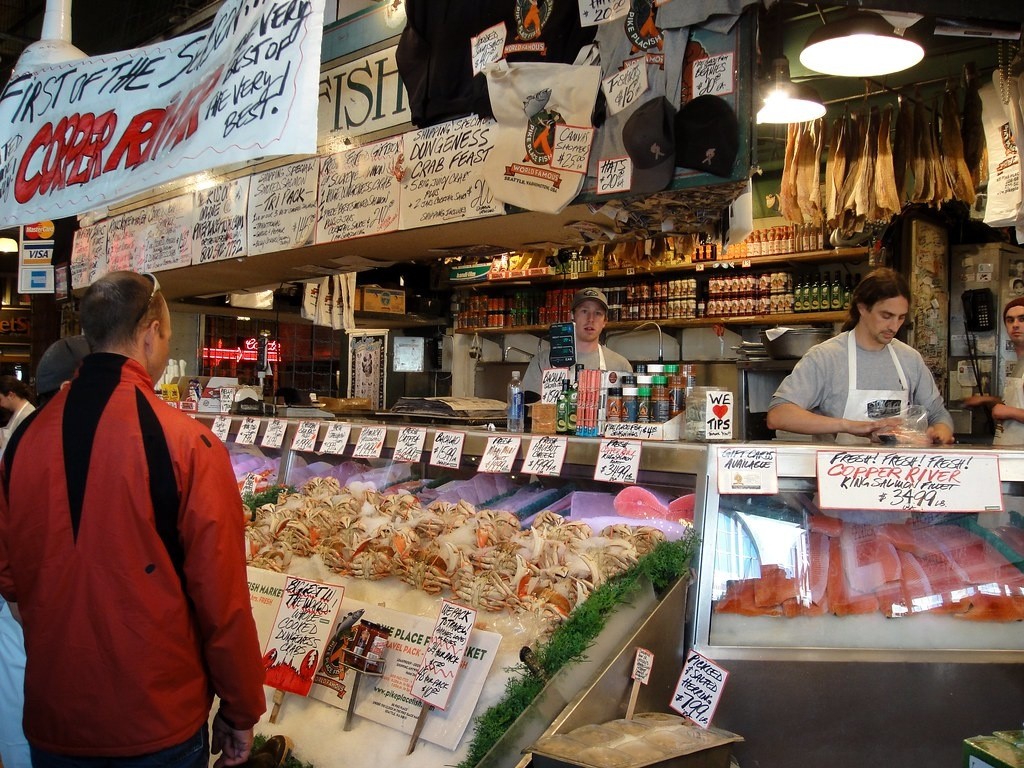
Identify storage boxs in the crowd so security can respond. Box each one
[230,291,274,309]
[354,287,406,313]
[449,251,551,282]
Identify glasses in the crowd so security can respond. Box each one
[135,272,161,322]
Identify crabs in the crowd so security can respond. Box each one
[242,477,665,632]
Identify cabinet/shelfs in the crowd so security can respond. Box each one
[452,245,892,333]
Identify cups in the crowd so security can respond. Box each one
[684,386,727,442]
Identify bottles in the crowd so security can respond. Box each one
[556,379,570,434]
[745,223,823,257]
[605,363,696,422]
[457,271,853,328]
[506,371,524,432]
[567,364,584,435]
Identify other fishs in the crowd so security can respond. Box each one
[777,87,988,245]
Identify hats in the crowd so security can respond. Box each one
[1003,297,1024,325]
[672,95,739,177]
[570,287,609,310]
[36,335,88,395]
[622,96,677,169]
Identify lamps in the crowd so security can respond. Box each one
[15,0,93,75]
[0,237,18,253]
[800,13,927,76]
[755,50,826,126]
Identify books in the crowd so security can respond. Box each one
[576,369,633,438]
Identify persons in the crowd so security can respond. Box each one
[767,268,956,445]
[518,288,634,427]
[992,294,1024,445]
[0,269,267,768]
[0,335,92,768]
